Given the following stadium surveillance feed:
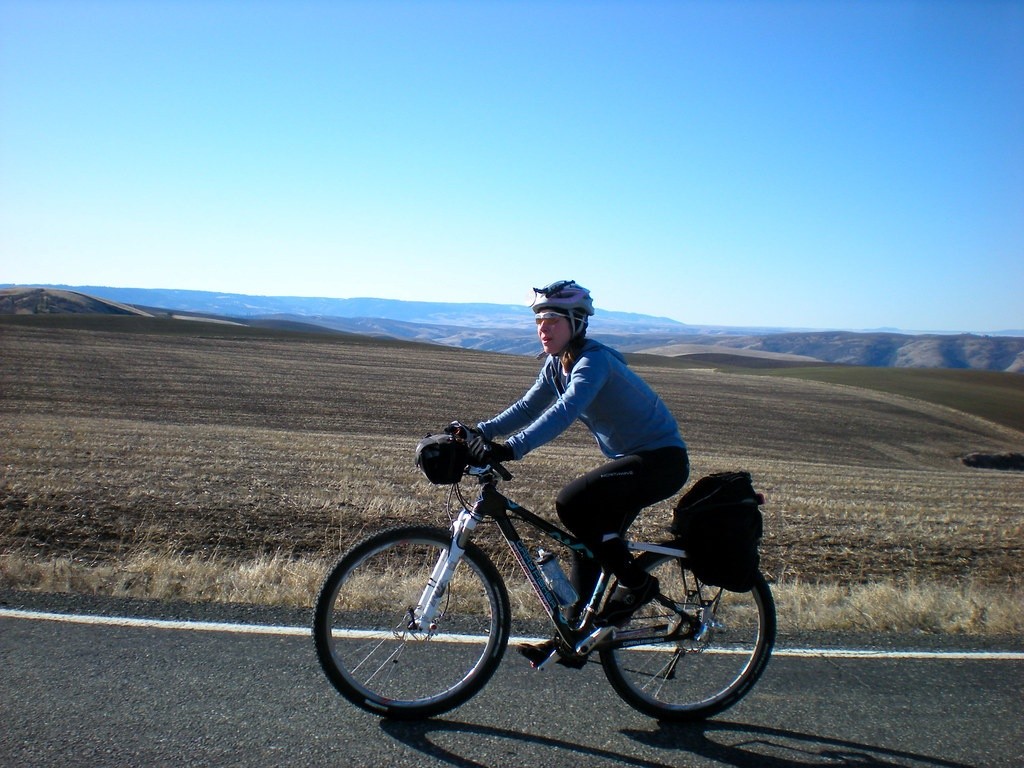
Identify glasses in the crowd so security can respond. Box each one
[534,312,587,325]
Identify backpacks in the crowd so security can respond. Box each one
[676,471,764,594]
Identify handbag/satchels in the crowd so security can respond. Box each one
[413,433,466,485]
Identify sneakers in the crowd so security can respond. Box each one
[516,640,589,670]
[592,572,659,628]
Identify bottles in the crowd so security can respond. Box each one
[536,549,580,608]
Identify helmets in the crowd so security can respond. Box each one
[528,280,595,324]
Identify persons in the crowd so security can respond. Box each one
[457,279,690,669]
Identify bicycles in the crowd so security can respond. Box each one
[314,419,777,725]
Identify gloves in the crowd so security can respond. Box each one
[443,425,484,440]
[469,435,515,468]
